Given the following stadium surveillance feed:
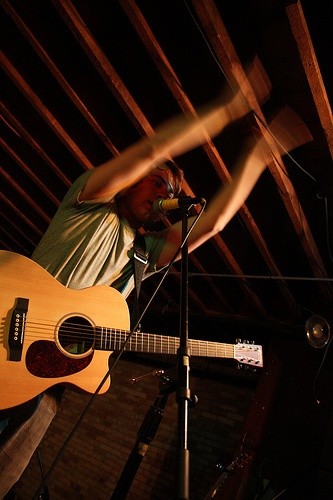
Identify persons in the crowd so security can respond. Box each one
[0,59,313,500]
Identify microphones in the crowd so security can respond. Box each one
[152,196,205,214]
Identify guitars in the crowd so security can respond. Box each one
[0,249,264,409]
[202,452,254,500]
